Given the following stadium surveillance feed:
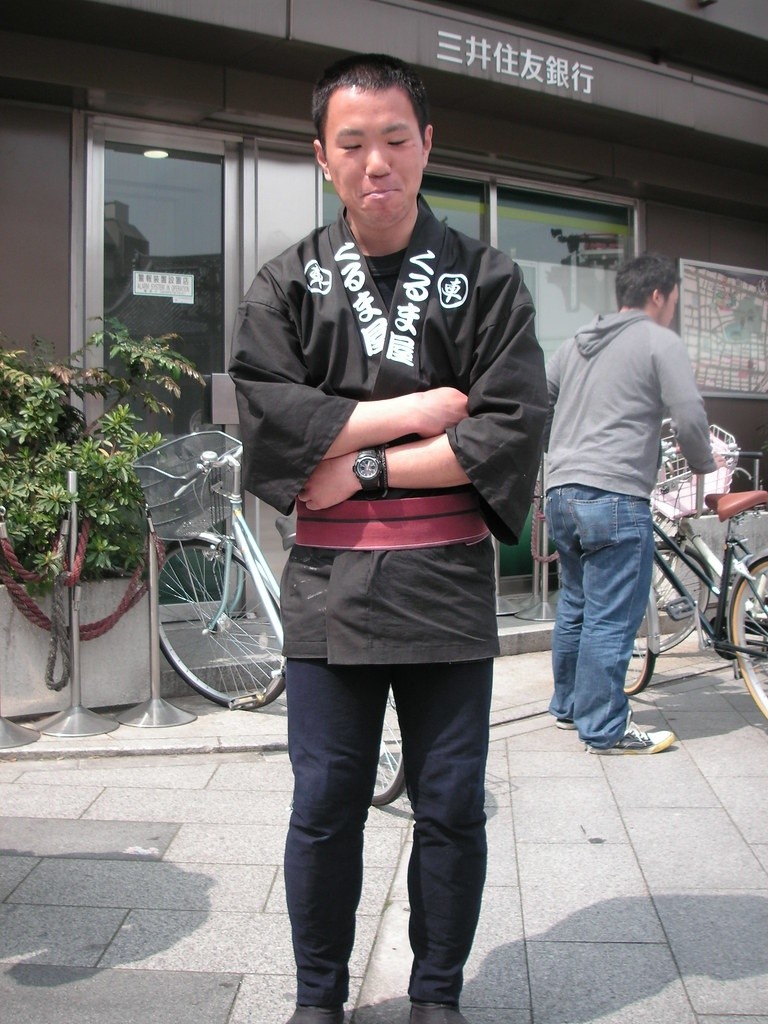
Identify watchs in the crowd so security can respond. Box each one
[354,446,384,500]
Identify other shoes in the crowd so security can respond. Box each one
[285,1003,344,1024]
[409,1001,470,1024]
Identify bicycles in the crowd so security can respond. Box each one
[130,430,404,807]
[631,422,768,673]
[624,418,767,721]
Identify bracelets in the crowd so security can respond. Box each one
[378,449,388,496]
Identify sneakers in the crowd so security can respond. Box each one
[584,710,675,755]
[556,717,577,730]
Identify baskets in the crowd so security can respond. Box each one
[651,424,738,521]
[132,431,245,540]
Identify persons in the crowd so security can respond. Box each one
[230,56,551,1024]
[546,252,726,755]
